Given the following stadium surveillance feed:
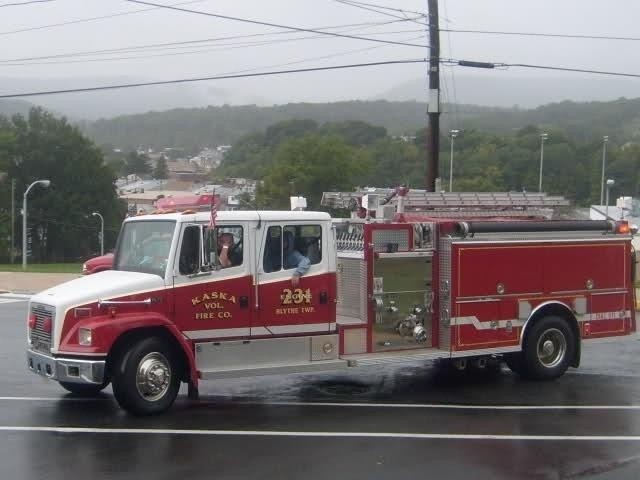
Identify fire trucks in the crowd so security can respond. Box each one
[23,189,636,417]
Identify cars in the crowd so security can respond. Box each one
[81,232,175,275]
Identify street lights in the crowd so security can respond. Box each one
[601,134,608,203]
[448,128,462,192]
[93,211,105,256]
[537,131,548,190]
[19,178,53,270]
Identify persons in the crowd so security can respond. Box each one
[217,232,241,268]
[267,229,311,289]
[357,207,377,232]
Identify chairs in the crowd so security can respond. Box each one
[296,238,319,261]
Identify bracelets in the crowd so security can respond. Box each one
[222,246,229,249]
